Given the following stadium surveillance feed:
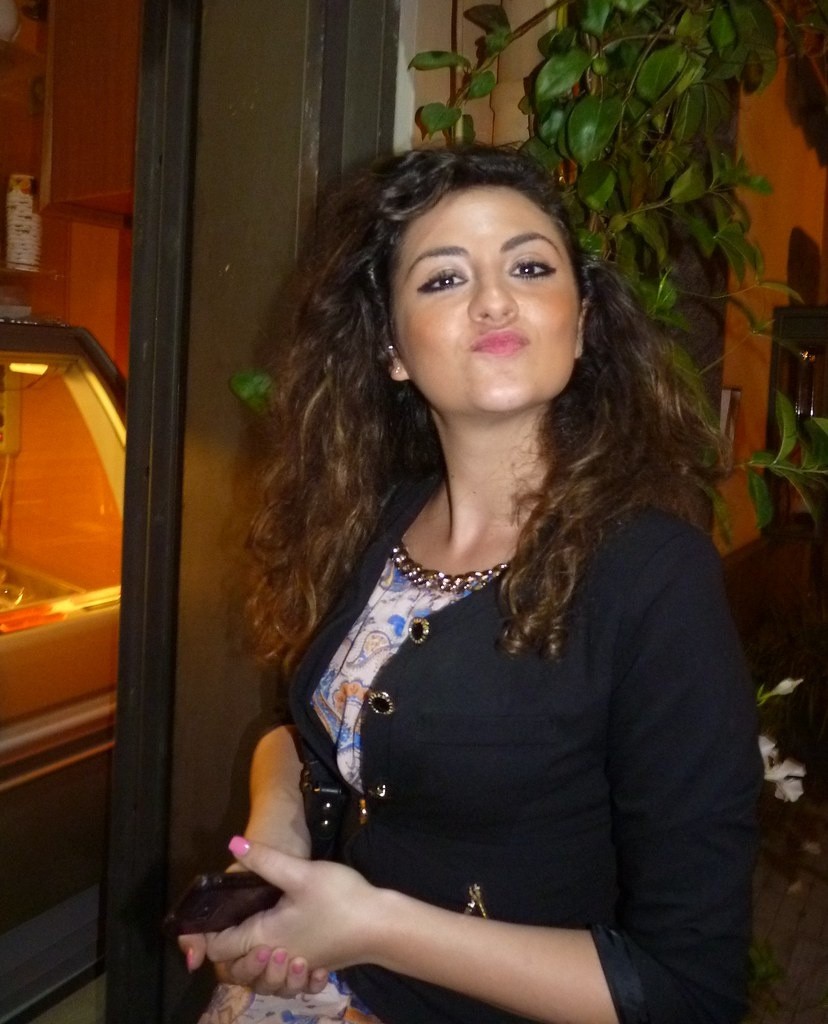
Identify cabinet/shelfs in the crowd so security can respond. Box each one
[37,0,144,230]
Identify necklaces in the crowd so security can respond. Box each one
[390,540,509,594]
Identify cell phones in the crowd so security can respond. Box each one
[165,873,284,935]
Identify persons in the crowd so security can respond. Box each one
[179,139,764,1024]
